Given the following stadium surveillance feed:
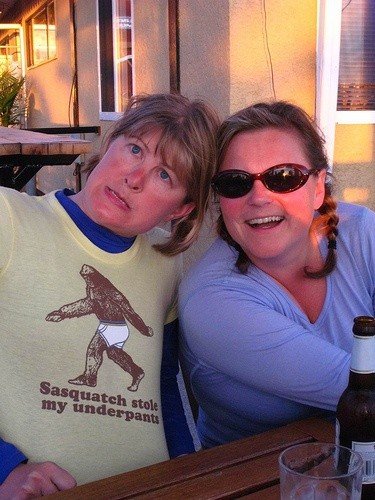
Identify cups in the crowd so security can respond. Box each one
[278,442,363,500]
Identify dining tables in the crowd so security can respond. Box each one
[31,415,337,500]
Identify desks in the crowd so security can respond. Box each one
[0,126,92,191]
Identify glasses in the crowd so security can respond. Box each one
[209,162,318,199]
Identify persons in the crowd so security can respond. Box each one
[178,99,375,452]
[1,90,223,500]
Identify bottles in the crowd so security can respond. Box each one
[335,315,375,500]
